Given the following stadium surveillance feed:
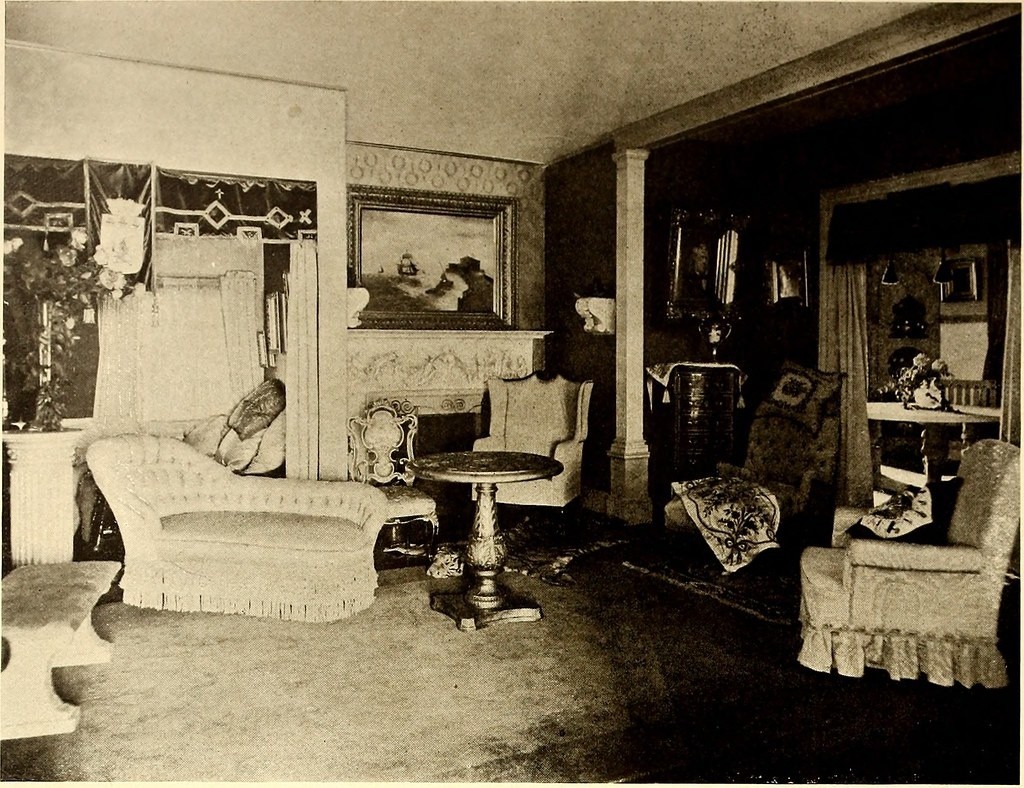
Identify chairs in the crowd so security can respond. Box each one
[470,371,594,557]
[344,398,440,576]
[664,412,841,568]
[940,379,999,461]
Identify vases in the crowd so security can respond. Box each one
[897,375,951,412]
[3,425,82,566]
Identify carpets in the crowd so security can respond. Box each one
[0,569,749,782]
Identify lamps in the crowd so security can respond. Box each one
[881,256,900,286]
[931,247,953,284]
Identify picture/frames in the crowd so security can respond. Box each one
[344,182,520,331]
[666,207,752,322]
[938,256,981,303]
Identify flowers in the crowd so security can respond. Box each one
[896,352,950,385]
[3,225,128,432]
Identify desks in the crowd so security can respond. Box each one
[404,450,564,633]
[863,402,1002,485]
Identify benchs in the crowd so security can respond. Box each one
[1,560,123,742]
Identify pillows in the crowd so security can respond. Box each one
[178,378,285,474]
[753,356,849,439]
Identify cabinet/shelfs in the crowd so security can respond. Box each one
[649,362,738,481]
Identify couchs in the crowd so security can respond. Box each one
[796,439,1021,688]
[85,433,389,624]
